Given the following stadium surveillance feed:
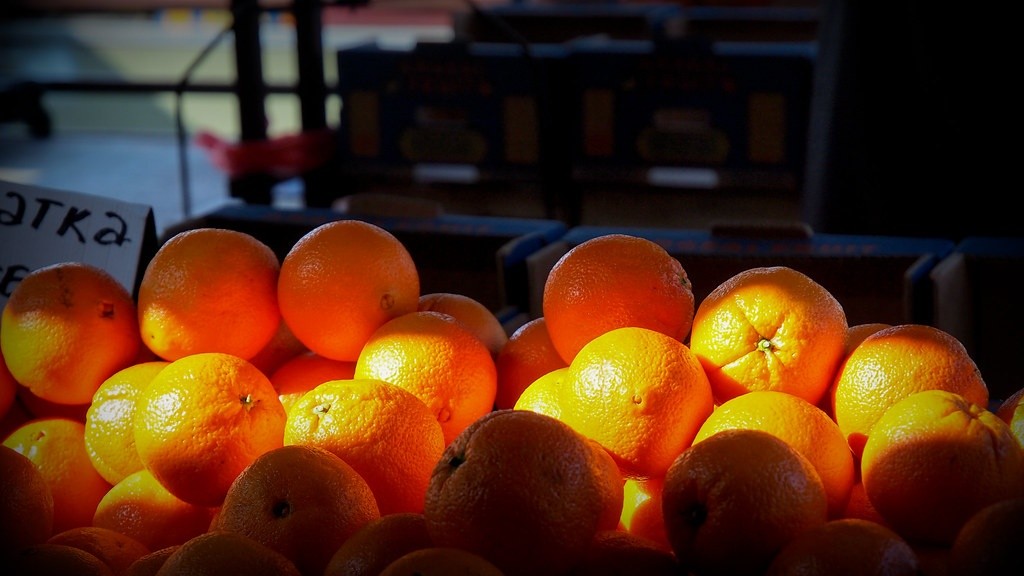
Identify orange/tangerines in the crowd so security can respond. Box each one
[4,220,1023,576]
